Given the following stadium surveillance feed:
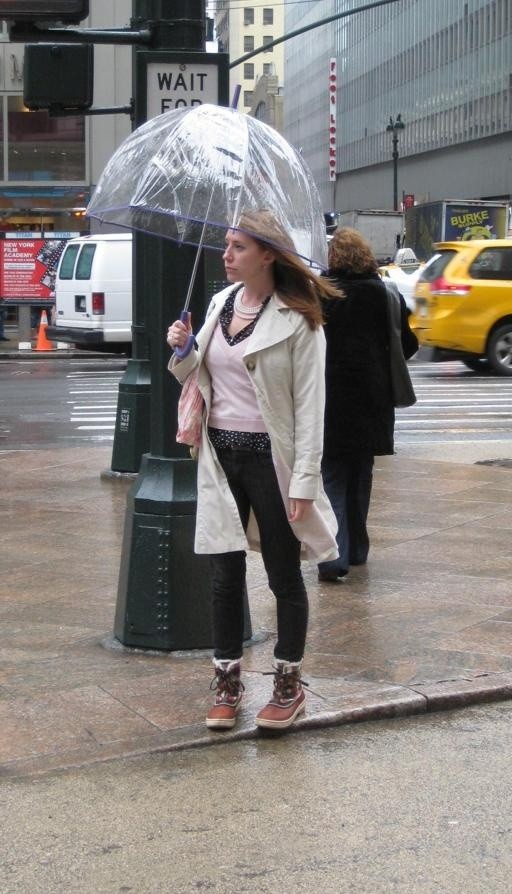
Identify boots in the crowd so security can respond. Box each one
[253,656,307,729]
[204,656,246,731]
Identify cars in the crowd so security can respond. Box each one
[406,236,512,377]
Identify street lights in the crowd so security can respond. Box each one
[386,113,406,212]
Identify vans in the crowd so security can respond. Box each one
[42,228,335,355]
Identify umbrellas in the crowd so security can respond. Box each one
[80,84,331,358]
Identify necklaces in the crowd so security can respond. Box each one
[234,284,263,321]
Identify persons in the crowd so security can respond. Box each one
[166,204,343,731]
[313,226,421,583]
[1,305,11,342]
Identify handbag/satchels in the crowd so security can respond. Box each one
[382,280,418,409]
[174,364,203,449]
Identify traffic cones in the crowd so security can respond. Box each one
[31,309,56,351]
